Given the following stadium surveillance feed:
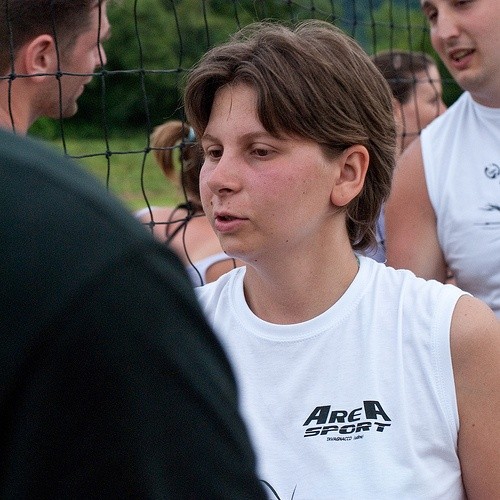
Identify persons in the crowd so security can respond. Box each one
[351,50,455,285]
[129,120,246,288]
[0,0,112,137]
[385,0,499,321]
[182,18,499,500]
